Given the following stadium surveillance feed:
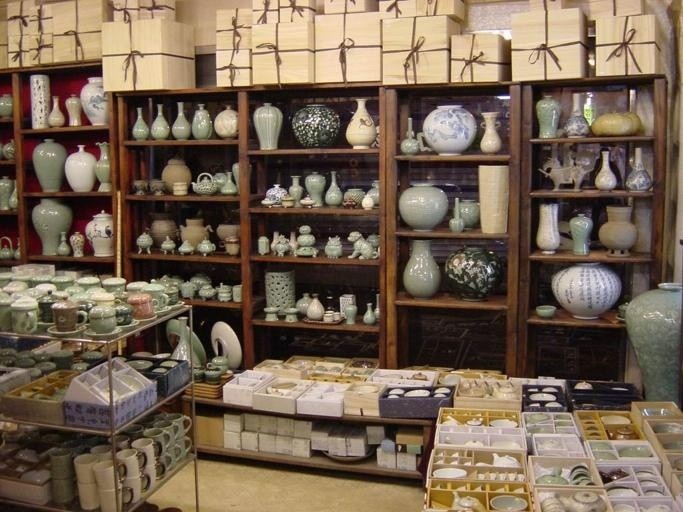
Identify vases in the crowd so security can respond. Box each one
[0,72,683,413]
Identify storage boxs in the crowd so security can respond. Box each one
[4,1,663,91]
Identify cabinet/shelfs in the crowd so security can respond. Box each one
[5,58,118,285]
[182,395,432,481]
[0,305,195,512]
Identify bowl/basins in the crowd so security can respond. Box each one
[536,305,557,319]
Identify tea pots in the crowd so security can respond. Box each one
[192,172,218,195]
[0,237,15,260]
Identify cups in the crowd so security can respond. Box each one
[47,408,195,512]
[0,270,185,340]
[428,370,682,512]
[173,182,188,196]
